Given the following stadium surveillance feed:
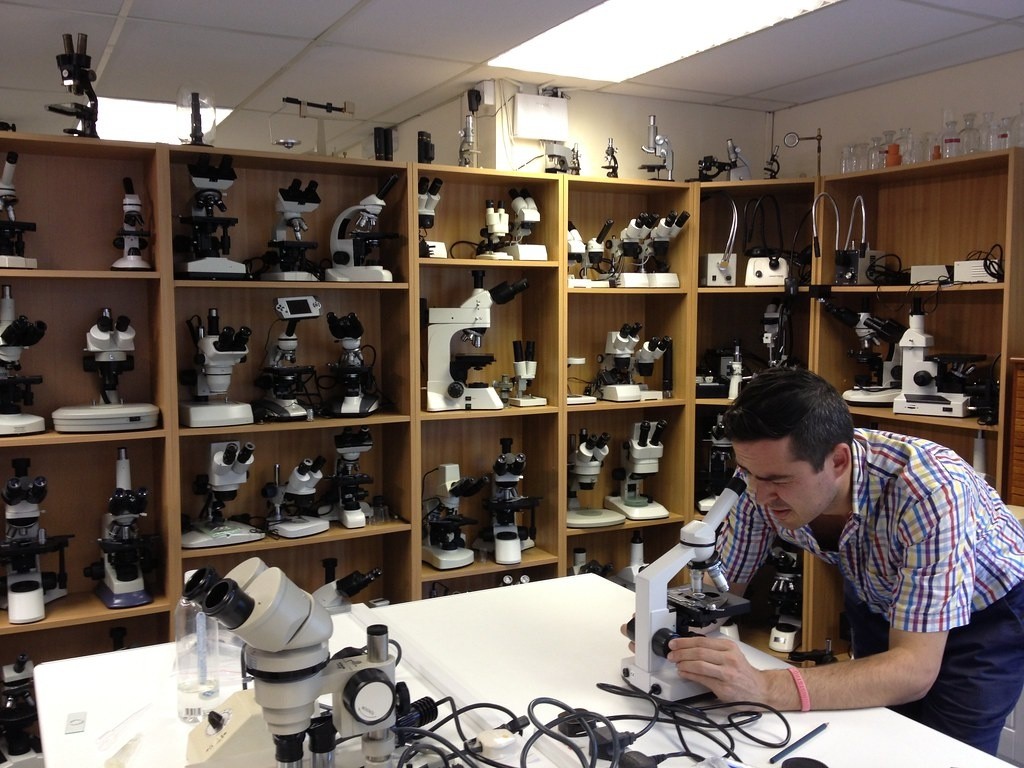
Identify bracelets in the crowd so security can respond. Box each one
[787,667,811,711]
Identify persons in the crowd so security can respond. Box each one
[621,366,1024,758]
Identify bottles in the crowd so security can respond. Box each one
[175,570,222,725]
[840,106,1024,174]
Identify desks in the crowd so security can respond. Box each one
[34,573,1019,768]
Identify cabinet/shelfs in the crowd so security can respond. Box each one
[563,174,694,591]
[163,143,417,640]
[807,147,1024,670]
[688,177,820,668]
[413,161,564,601]
[0,136,178,642]
